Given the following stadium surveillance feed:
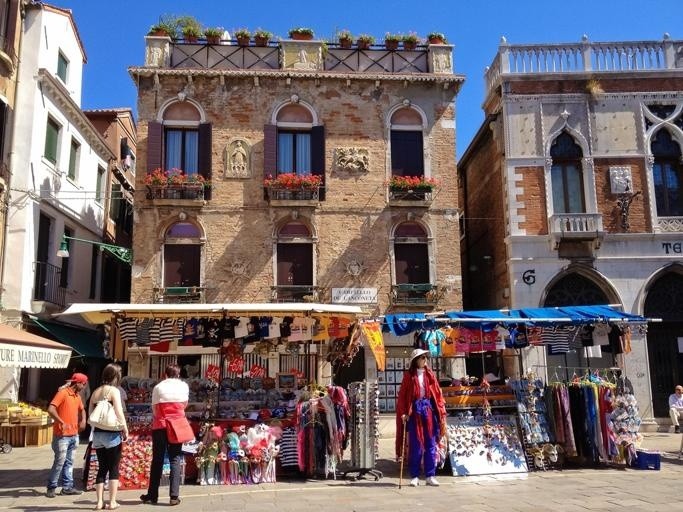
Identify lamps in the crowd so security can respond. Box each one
[56,241,70,258]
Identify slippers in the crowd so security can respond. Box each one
[94,502,120,509]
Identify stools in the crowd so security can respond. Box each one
[637,450,662,470]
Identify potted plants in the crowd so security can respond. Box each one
[427,31,446,44]
[254,29,273,47]
[177,16,202,43]
[385,32,401,49]
[404,32,418,48]
[357,33,374,48]
[335,30,354,48]
[288,25,314,41]
[151,23,172,37]
[204,28,221,45]
[232,29,251,47]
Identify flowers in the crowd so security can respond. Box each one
[263,173,323,189]
[141,167,205,186]
[384,175,440,190]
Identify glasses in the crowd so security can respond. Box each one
[417,356,428,360]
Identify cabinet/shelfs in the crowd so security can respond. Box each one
[116,375,307,486]
[435,384,562,473]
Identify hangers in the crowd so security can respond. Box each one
[548,365,623,386]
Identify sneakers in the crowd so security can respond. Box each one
[45,487,56,498]
[169,496,181,505]
[426,477,440,487]
[140,494,157,504]
[60,488,82,495]
[675,426,680,433]
[411,478,420,487]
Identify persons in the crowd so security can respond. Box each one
[140,364,189,505]
[58,366,91,445]
[113,377,128,412]
[86,362,130,511]
[45,373,88,498]
[668,385,683,434]
[395,348,447,487]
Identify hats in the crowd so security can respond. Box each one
[408,348,429,363]
[65,373,89,384]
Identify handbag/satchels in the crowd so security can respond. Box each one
[88,399,124,432]
[164,416,194,443]
